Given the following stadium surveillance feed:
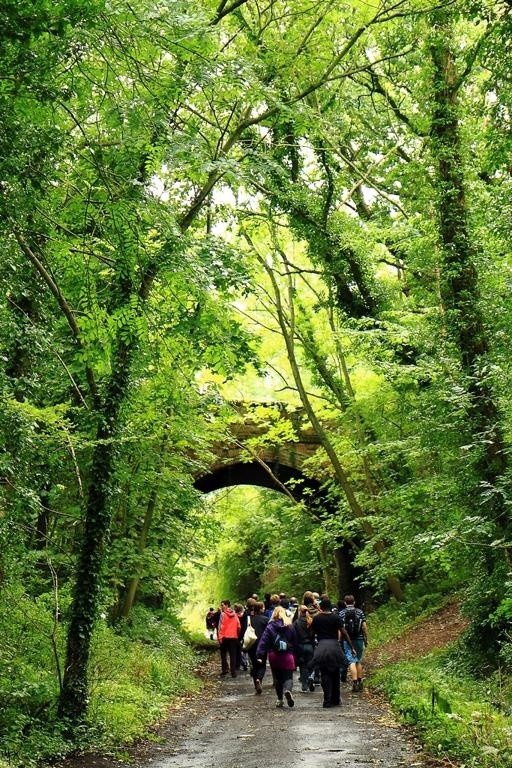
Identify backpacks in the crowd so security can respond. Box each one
[272,633,289,653]
[344,609,361,639]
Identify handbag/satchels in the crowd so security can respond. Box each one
[241,625,258,651]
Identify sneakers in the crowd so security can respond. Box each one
[221,671,228,675]
[255,679,262,694]
[353,678,363,692]
[285,690,294,707]
[232,671,236,678]
[276,700,283,707]
[308,678,314,691]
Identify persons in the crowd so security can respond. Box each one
[203,591,368,693]
[256,600,301,709]
[311,600,356,708]
